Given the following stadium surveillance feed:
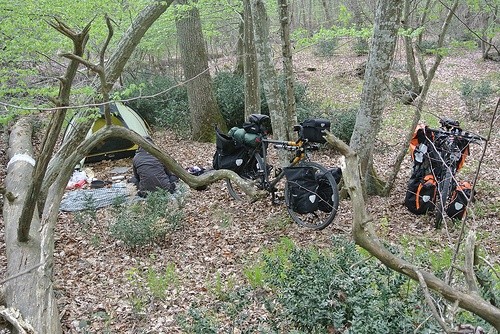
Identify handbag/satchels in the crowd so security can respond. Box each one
[299,119,331,145]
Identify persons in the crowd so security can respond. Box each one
[127,135,180,198]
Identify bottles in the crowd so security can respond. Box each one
[415,139,429,163]
[75,179,87,187]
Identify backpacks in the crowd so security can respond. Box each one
[211,123,249,175]
[445,181,477,221]
[401,173,438,214]
[313,167,342,212]
[283,166,320,214]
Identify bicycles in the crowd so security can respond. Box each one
[226,112,339,230]
[408,120,486,230]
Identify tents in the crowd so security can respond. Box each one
[61,97,155,171]
